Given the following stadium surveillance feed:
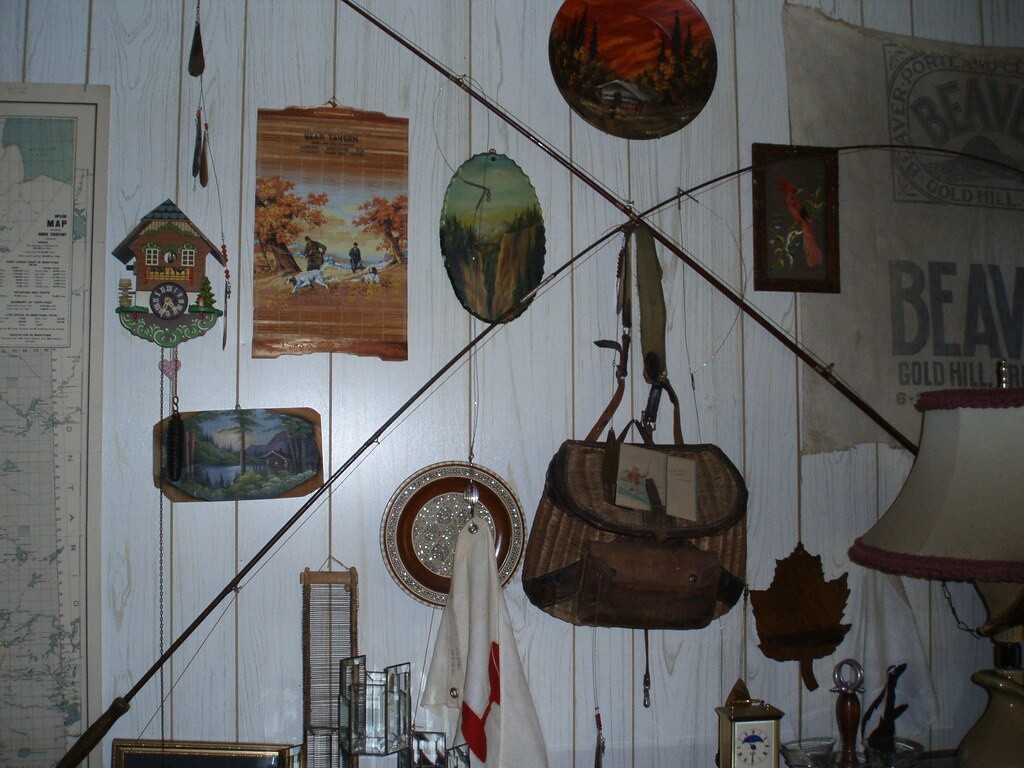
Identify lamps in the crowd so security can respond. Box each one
[848,357,1024,768]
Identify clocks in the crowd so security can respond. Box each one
[714,704,786,768]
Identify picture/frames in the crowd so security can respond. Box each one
[751,140,840,294]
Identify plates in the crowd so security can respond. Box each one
[379,461,529,610]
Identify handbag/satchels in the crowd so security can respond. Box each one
[520,227,748,632]
[579,536,720,630]
